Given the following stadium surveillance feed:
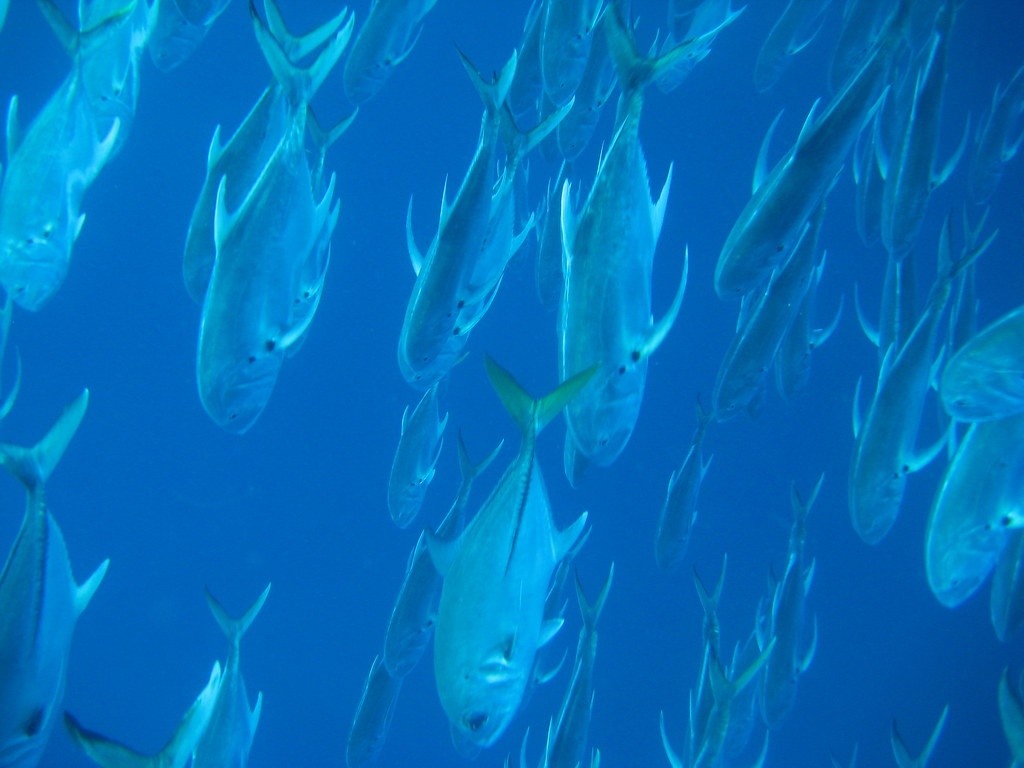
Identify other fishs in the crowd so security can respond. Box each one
[0,1,1024,767]
